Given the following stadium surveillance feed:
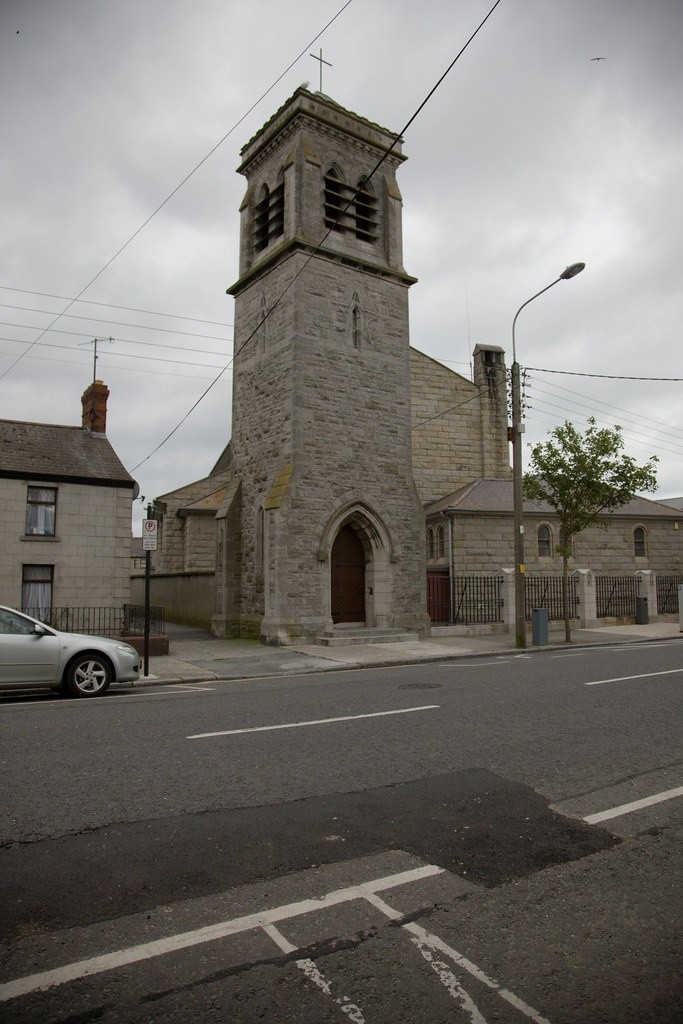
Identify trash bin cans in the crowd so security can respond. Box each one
[636,597,649,624]
[533,608,549,645]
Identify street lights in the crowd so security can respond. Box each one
[511,260,588,647]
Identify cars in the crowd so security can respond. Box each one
[0,606,141,697]
